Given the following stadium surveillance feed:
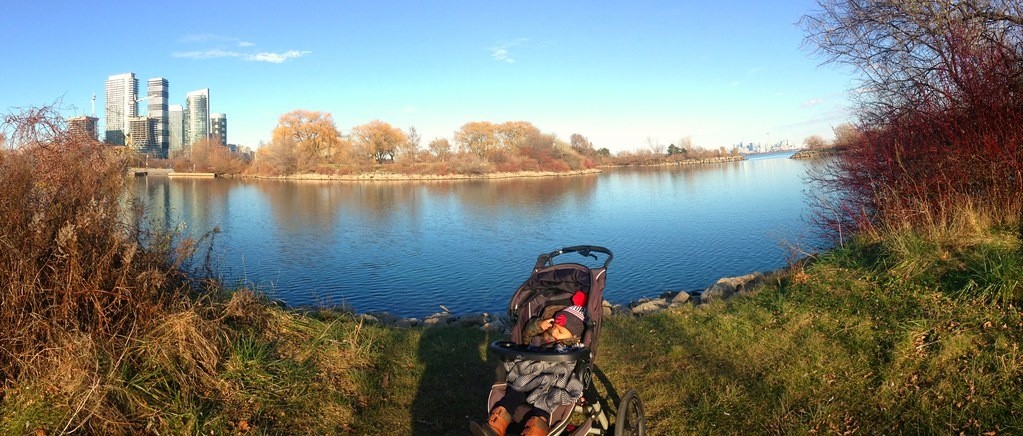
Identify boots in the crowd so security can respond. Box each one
[470,406,512,436]
[520,416,549,436]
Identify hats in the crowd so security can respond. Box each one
[555,291,586,338]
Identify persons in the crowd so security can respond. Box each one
[469,306,589,436]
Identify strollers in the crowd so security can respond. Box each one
[467,243,647,436]
